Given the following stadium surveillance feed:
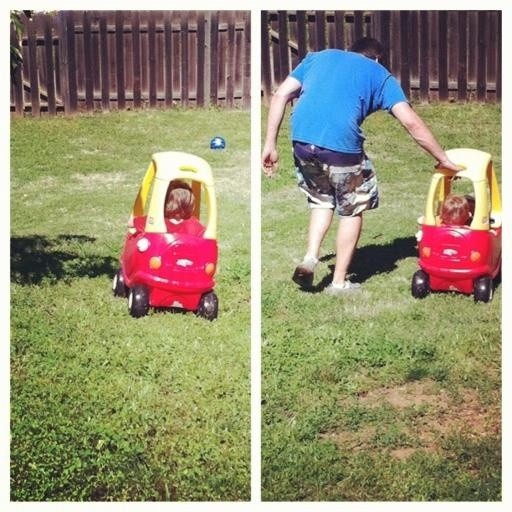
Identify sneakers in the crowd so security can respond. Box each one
[292,259,315,290]
[325,279,362,297]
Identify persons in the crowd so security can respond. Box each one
[127,183,207,237]
[260,35,466,291]
[110,149,218,322]
[438,195,473,228]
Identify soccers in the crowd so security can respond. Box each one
[211,137,226,150]
